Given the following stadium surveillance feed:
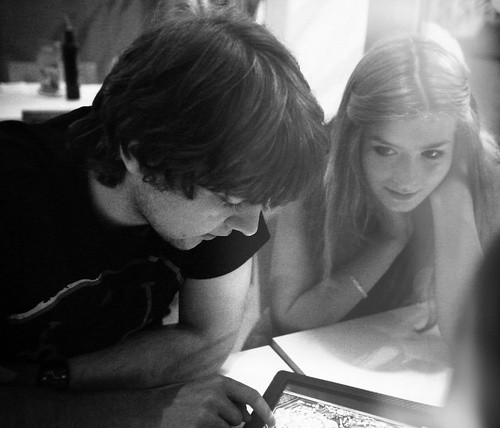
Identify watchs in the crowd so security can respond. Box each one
[35,357,70,393]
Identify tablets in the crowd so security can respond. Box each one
[241,370,444,428]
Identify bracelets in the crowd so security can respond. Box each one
[343,268,368,298]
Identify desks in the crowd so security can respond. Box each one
[221,301,454,409]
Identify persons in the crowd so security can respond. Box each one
[0,0,332,428]
[244,34,500,354]
